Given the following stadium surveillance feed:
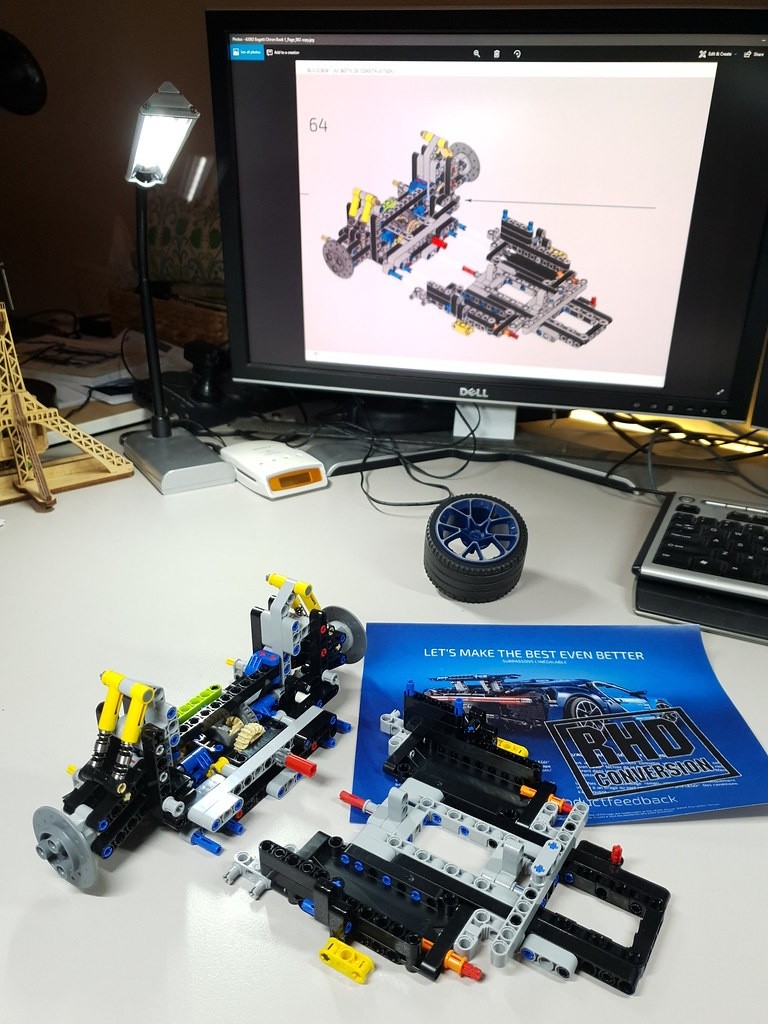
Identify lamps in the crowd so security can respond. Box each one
[119,73,241,493]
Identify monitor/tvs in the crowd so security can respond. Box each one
[203,7,768,495]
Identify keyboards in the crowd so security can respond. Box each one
[631,491,768,647]
